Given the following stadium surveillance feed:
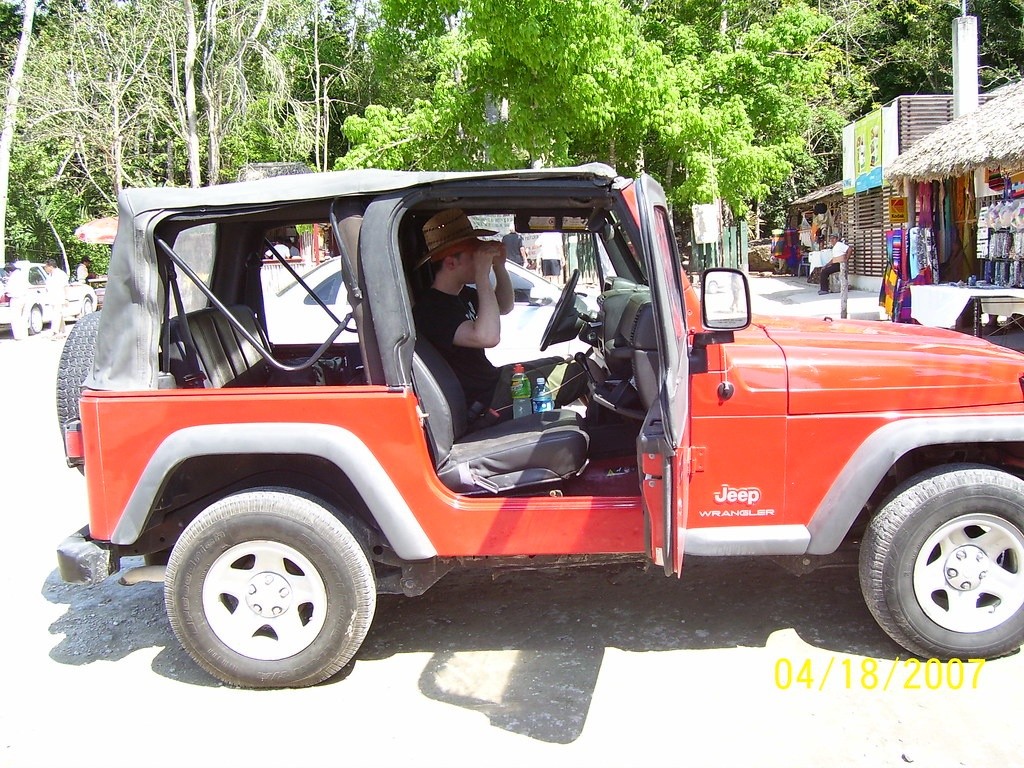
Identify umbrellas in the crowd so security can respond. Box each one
[74,216,119,245]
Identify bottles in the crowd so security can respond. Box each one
[532,377,553,414]
[510,363,533,419]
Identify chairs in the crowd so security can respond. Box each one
[413,327,591,496]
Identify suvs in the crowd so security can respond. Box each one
[54,137,1024,692]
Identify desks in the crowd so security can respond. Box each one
[910,283,1024,338]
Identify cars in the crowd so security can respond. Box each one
[264,240,598,369]
[0,261,110,341]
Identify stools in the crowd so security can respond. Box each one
[828,271,841,293]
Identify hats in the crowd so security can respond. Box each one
[413,208,499,273]
[829,233,838,238]
[430,237,503,263]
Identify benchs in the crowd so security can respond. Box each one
[159,303,347,388]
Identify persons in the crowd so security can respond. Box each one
[4,264,32,341]
[263,237,299,260]
[502,220,565,287]
[411,206,592,426]
[75,256,97,284]
[45,259,68,340]
[818,234,852,296]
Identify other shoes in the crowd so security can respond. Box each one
[818,289,829,295]
[984,325,1007,336]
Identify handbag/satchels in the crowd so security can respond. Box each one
[265,357,352,387]
